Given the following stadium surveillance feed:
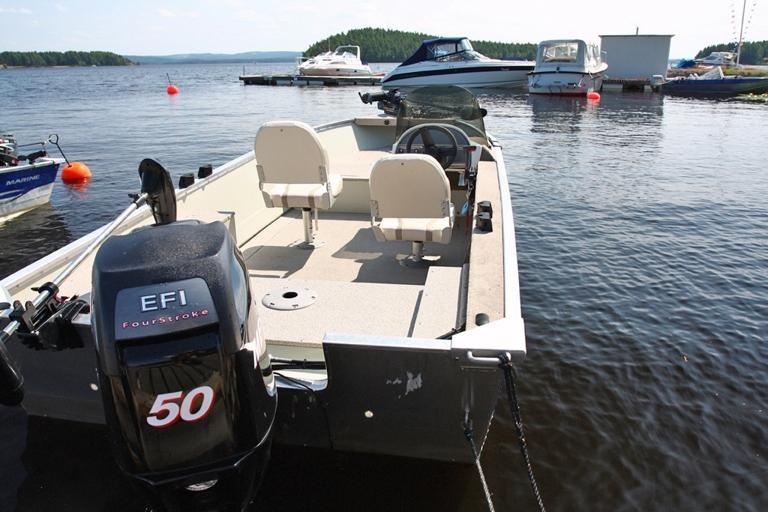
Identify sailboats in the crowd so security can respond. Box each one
[686,0,756,70]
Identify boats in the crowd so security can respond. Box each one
[0,84,527,512]
[379,37,535,89]
[0,133,66,226]
[527,39,608,95]
[651,75,767,96]
[295,40,388,76]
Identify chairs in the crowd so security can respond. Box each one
[368,154,456,268]
[254,121,344,250]
